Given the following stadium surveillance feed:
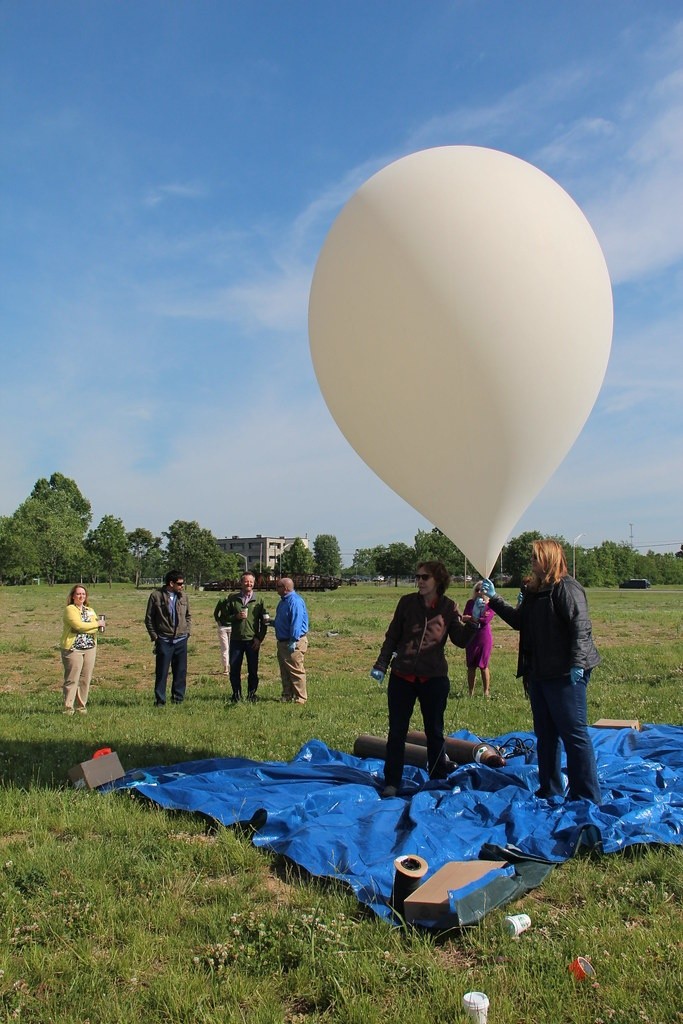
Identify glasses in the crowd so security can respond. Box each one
[416,574,433,582]
[277,585,283,588]
[175,582,184,586]
[531,554,539,560]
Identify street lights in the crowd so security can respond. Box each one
[573,533,588,579]
[236,553,247,571]
[280,542,294,579]
[630,522,634,549]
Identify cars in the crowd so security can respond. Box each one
[373,575,385,582]
[466,576,472,581]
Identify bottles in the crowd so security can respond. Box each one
[99,615,105,633]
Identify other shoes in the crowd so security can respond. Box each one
[381,786,399,799]
[534,788,554,802]
[294,696,305,705]
[230,695,243,704]
[247,695,258,700]
[279,695,291,703]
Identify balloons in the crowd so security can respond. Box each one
[308,145,613,579]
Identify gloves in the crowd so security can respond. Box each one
[370,669,386,684]
[481,579,495,599]
[473,597,485,619]
[568,666,585,686]
[288,642,297,653]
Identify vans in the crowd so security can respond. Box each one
[619,579,650,589]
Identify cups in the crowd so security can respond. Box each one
[463,992,490,1024]
[503,914,531,936]
[569,957,596,981]
[241,606,248,619]
[263,612,270,625]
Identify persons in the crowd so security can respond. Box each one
[460,581,494,698]
[61,585,105,715]
[145,571,191,705]
[263,578,309,704]
[224,572,269,702]
[481,540,603,806]
[214,594,237,676]
[371,561,485,796]
